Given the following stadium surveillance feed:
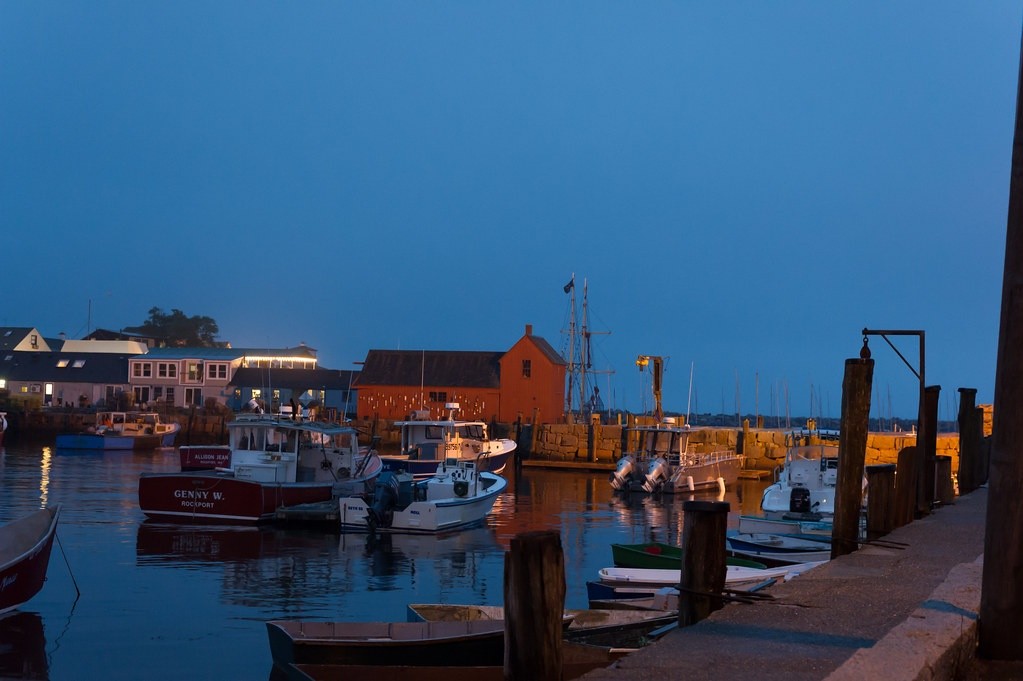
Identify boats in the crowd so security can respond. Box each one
[379,403,518,474]
[759,390,868,521]
[265,513,870,681]
[2,501,63,616]
[609,362,746,494]
[55,410,182,450]
[136,375,382,527]
[337,450,509,533]
